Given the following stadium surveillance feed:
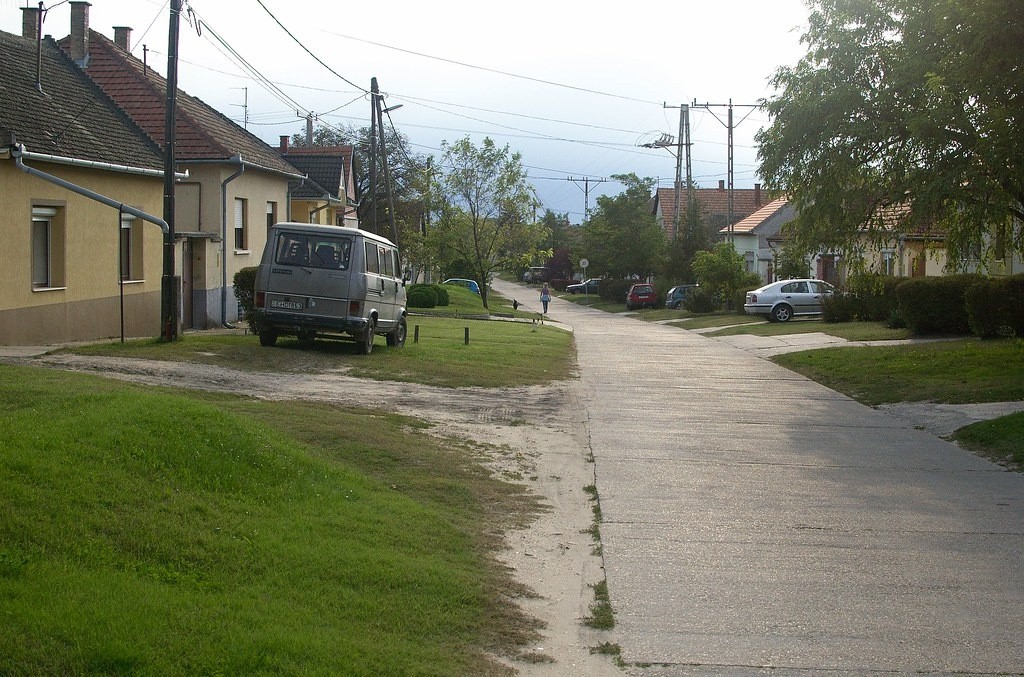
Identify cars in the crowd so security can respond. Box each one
[743,277,841,322]
[626,284,659,308]
[566,278,606,295]
[667,287,706,308]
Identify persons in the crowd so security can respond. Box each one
[540,282,551,314]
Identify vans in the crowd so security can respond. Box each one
[441,278,480,295]
[523,267,551,284]
[253,222,407,349]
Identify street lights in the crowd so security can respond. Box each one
[372,77,405,242]
